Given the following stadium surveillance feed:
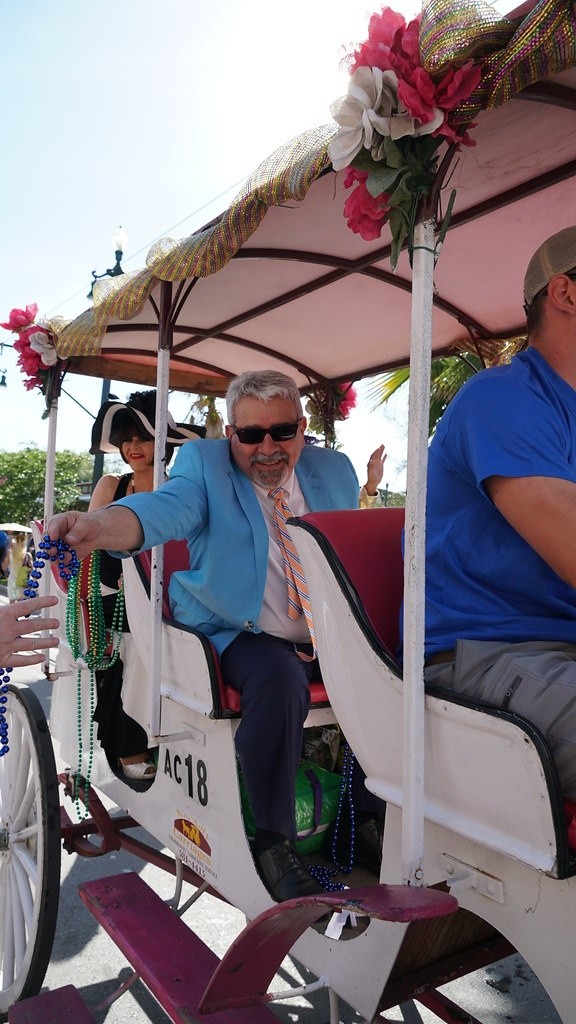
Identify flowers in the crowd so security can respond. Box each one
[0,302,63,420]
[304,382,358,451]
[326,5,484,274]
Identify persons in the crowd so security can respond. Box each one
[41,370,361,902]
[88,390,208,780]
[401,229,575,796]
[360,444,387,508]
[0,530,37,605]
[0,596,60,668]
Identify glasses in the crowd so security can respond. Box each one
[230,418,300,444]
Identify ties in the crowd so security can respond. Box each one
[268,487,318,662]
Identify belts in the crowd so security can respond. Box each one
[424,651,455,666]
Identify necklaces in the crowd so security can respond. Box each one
[132,475,135,494]
[67,550,125,820]
[332,744,354,873]
[0,536,80,760]
[307,864,344,893]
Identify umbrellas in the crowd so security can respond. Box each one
[0,523,32,533]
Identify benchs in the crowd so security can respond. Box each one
[30,519,113,671]
[122,540,338,732]
[285,508,576,880]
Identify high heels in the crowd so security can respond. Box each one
[117,756,157,780]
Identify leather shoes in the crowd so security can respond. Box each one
[347,819,383,873]
[252,843,324,903]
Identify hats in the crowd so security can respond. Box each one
[523,226,576,315]
[89,389,207,455]
